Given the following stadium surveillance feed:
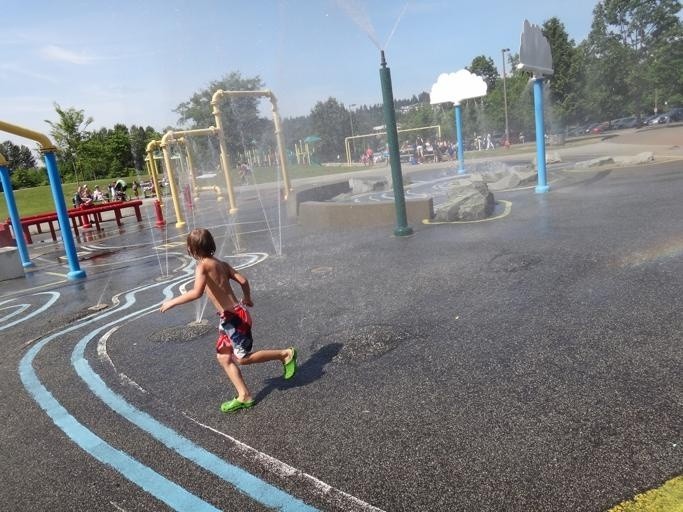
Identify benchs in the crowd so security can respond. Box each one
[386,154,435,166]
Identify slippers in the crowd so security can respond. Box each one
[221,394,256,413]
[283,346,297,381]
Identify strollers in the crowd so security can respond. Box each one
[112,179,130,202]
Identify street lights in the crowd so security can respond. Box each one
[501,46,511,146]
[339,101,358,163]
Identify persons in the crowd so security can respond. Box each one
[159,229,297,412]
[237,163,250,186]
[361,143,390,166]
[400,135,456,164]
[475,131,495,151]
[75,178,171,205]
